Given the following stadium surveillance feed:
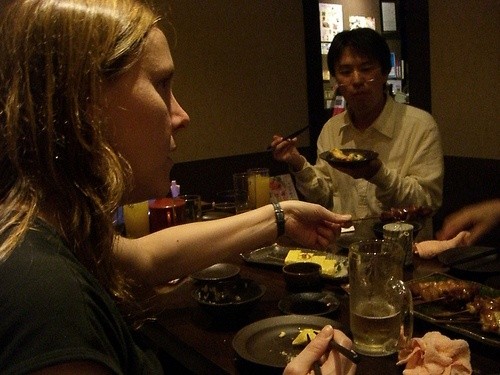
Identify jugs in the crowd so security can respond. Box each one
[382,221,414,265]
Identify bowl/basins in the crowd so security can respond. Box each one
[370,220,423,240]
[190,278,267,319]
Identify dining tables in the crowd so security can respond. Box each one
[128,204,500,375]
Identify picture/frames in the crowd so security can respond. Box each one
[378,0,399,34]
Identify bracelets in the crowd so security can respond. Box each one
[273,203,286,241]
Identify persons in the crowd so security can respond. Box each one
[270,28,443,243]
[0,0,355,375]
[437,200,500,247]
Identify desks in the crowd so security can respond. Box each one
[168,146,500,290]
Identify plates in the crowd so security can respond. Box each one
[238,241,348,283]
[232,315,354,367]
[277,292,340,315]
[192,263,240,280]
[319,149,379,168]
[407,271,500,349]
[437,246,500,276]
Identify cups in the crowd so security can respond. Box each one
[348,239,404,357]
[282,262,322,291]
[122,167,270,239]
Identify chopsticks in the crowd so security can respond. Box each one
[306,331,358,375]
[267,123,309,153]
[443,248,500,268]
